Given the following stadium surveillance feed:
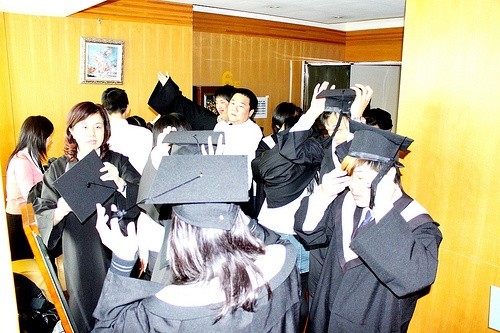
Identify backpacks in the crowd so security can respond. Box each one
[12,271,60,333]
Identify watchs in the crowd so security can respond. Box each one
[352,116,367,124]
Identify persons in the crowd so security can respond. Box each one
[6,70,444,332]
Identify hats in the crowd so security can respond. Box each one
[145,155,248,230]
[349,119,414,163]
[316,89,372,112]
[162,130,225,155]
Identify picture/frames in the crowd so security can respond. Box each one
[80,37,125,84]
